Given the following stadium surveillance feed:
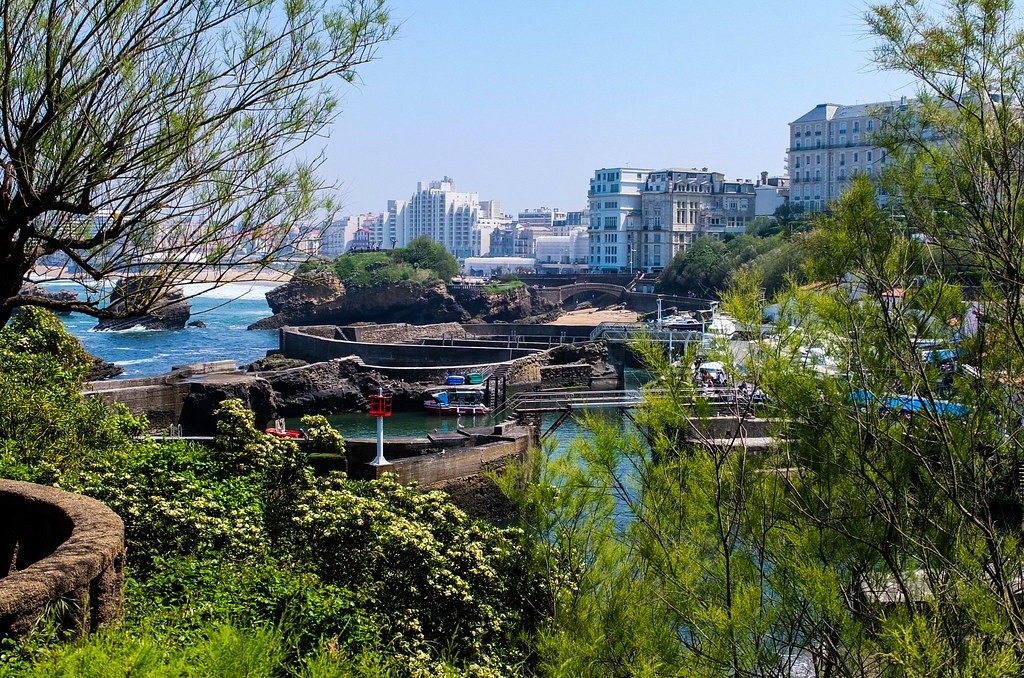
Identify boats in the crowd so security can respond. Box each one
[265,419,310,439]
[423,383,490,415]
[642,269,1024,423]
